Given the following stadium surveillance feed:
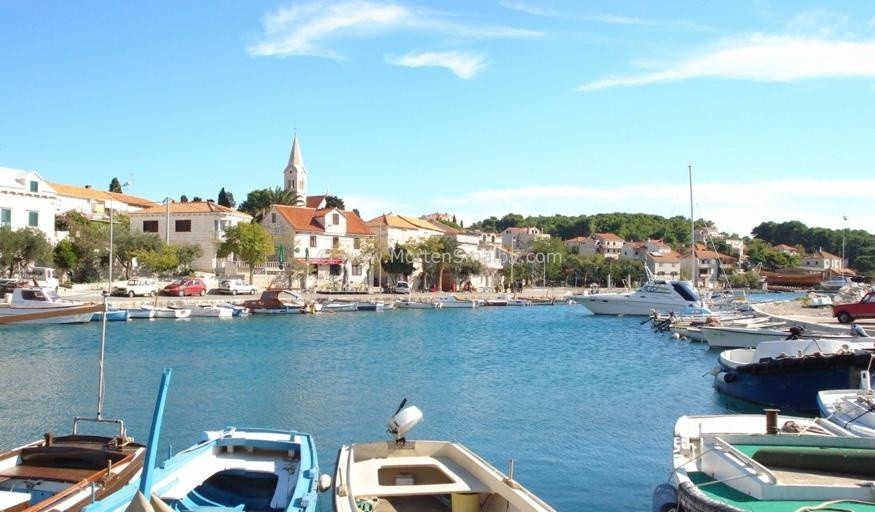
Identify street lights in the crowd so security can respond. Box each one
[109,182,132,296]
[162,196,177,247]
[511,234,519,283]
[376,211,395,293]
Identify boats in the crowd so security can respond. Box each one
[569,164,821,350]
[1,276,601,324]
[714,338,875,410]
[814,370,875,436]
[331,394,562,512]
[674,414,875,512]
[78,366,324,512]
[0,291,150,512]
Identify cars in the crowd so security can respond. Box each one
[833,291,875,324]
[825,273,848,291]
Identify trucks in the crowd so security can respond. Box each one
[23,267,63,291]
